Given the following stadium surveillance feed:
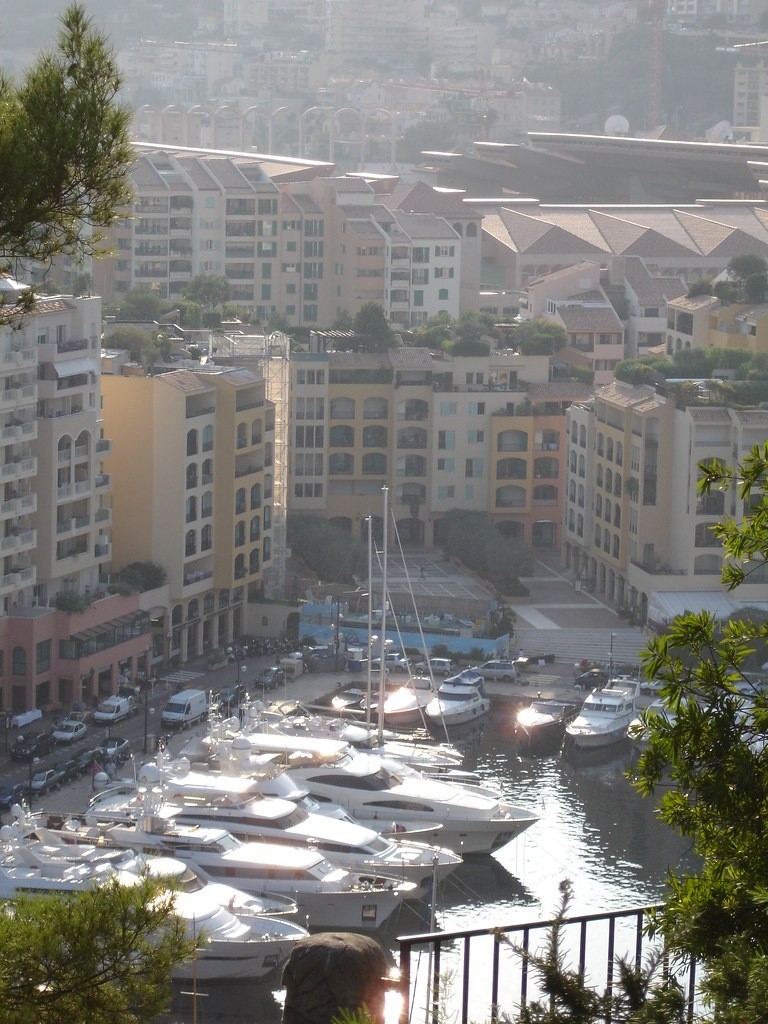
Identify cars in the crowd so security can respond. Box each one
[372,653,413,673]
[49,719,86,745]
[468,657,529,682]
[699,669,768,760]
[415,657,453,677]
[216,687,238,708]
[640,678,670,695]
[24,765,67,796]
[574,668,618,690]
[63,745,105,776]
[0,782,29,811]
[10,729,55,761]
[96,735,130,761]
[255,664,286,690]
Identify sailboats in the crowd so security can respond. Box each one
[0,487,540,987]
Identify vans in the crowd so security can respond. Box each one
[93,696,131,726]
[161,689,206,731]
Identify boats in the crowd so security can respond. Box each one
[332,688,366,714]
[627,692,698,743]
[383,667,441,726]
[424,664,492,727]
[359,688,391,714]
[564,664,642,748]
[514,685,585,738]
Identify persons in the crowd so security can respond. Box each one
[419,564,426,581]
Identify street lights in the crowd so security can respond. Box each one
[608,632,619,679]
[135,680,155,754]
[329,594,345,672]
[225,643,248,693]
[17,734,34,810]
